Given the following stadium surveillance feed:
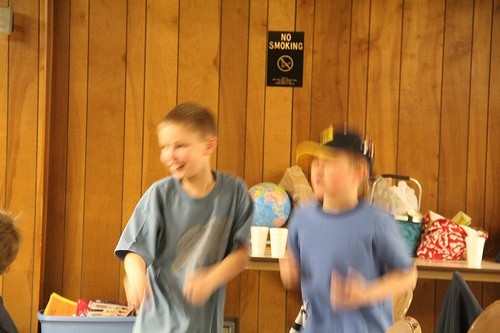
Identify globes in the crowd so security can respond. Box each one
[247,182,292,244]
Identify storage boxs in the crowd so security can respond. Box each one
[37,311,135,333]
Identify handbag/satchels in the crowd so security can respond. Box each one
[416,211,466,261]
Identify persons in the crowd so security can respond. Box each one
[277,132,418,333]
[0,212,20,333]
[114,103,254,333]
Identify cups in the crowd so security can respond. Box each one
[270,227,288,258]
[465,236,485,268]
[250,226,269,257]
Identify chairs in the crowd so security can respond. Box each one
[385,270,500,333]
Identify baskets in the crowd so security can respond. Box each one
[371,174,423,257]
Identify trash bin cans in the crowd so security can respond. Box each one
[38,310,135,333]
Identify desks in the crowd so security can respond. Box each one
[417,257,500,283]
